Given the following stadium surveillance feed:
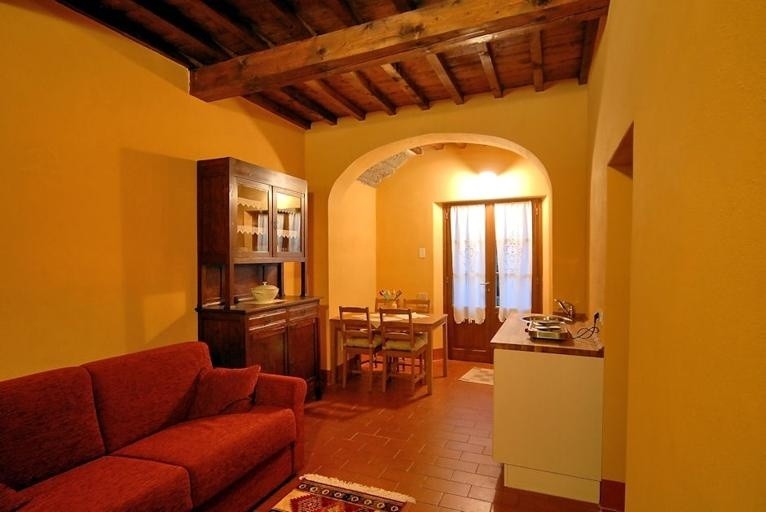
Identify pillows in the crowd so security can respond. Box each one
[187,364,261,421]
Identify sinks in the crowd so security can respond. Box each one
[521,315,575,325]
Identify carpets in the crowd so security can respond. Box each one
[253,472,417,512]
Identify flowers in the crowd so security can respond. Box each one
[376,288,403,302]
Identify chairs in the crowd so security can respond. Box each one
[339,298,431,392]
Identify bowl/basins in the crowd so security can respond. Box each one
[249,288,280,302]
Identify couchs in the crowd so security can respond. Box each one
[1,341,307,511]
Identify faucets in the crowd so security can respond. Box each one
[553,299,575,318]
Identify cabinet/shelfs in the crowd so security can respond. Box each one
[199,158,322,402]
